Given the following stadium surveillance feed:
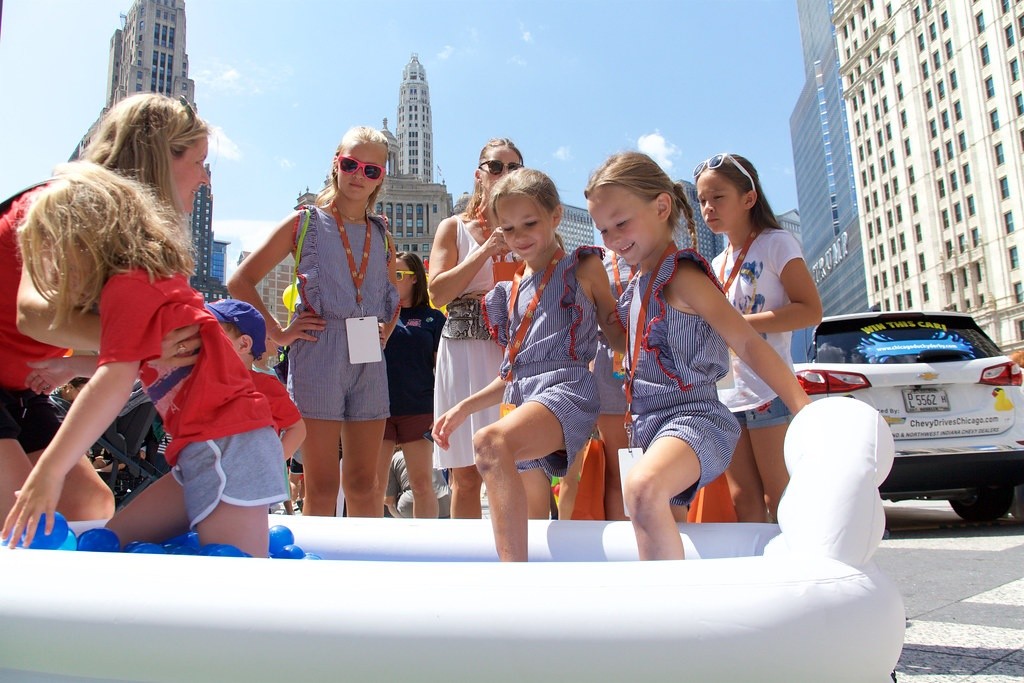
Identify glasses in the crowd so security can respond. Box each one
[692,153,757,193]
[479,160,524,174]
[336,156,386,182]
[395,270,415,282]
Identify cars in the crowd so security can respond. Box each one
[784,311,1024,525]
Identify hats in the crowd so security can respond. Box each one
[205,297,268,361]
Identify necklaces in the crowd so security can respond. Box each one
[340,213,365,222]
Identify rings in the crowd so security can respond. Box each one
[177,343,187,355]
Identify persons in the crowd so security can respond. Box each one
[384,444,452,522]
[48,377,90,425]
[682,153,822,524]
[0,89,211,542]
[431,168,628,562]
[429,137,557,521]
[593,241,641,522]
[367,248,450,522]
[582,149,815,562]
[287,443,304,511]
[229,125,400,519]
[202,298,307,465]
[2,159,290,559]
[250,337,281,383]
